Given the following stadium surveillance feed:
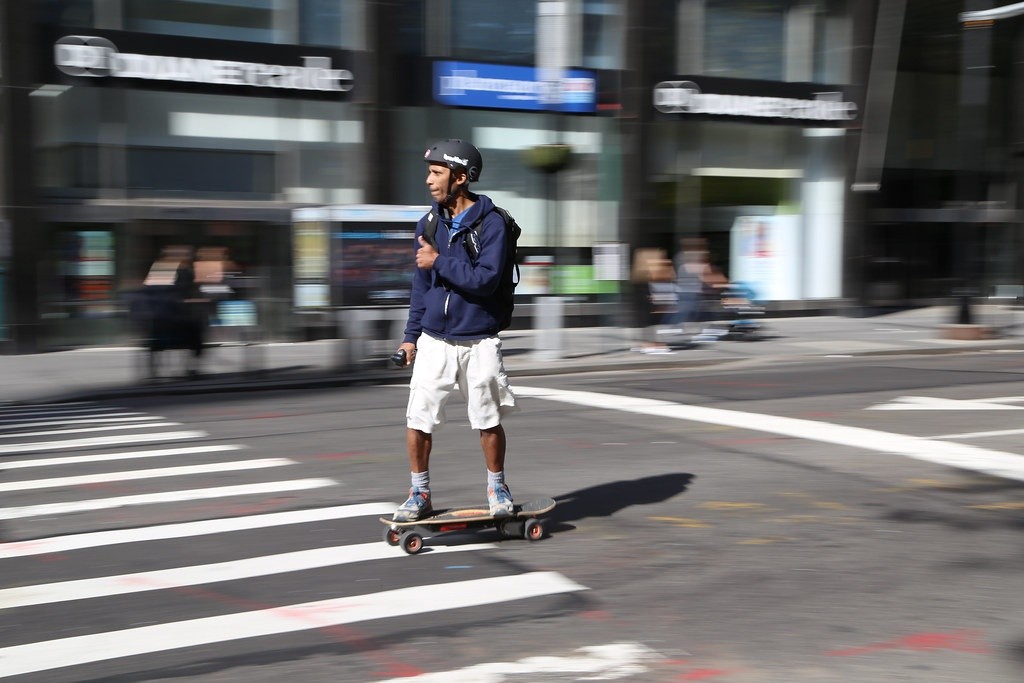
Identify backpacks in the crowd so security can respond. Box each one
[422,203,521,328]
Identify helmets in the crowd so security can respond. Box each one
[424,140,483,183]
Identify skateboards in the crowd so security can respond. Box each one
[379,496,556,554]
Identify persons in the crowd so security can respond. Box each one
[388,140,521,523]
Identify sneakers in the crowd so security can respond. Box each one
[486,483,514,515]
[392,487,433,523]
